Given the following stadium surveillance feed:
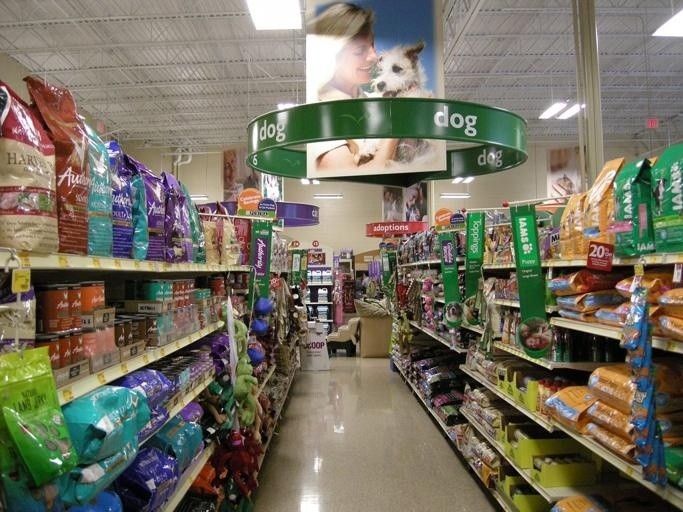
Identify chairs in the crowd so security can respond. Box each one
[326,316,359,358]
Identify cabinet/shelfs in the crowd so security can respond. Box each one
[388,248,683,512]
[0,243,305,512]
[303,281,335,340]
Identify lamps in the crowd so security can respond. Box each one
[313,179,344,200]
[440,176,474,199]
[649,0,682,39]
[536,69,585,120]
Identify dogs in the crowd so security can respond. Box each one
[351,41,434,168]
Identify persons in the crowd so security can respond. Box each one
[307,5,416,174]
[384,181,428,222]
[520,321,554,350]
[223,150,244,201]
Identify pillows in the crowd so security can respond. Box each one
[353,296,393,318]
